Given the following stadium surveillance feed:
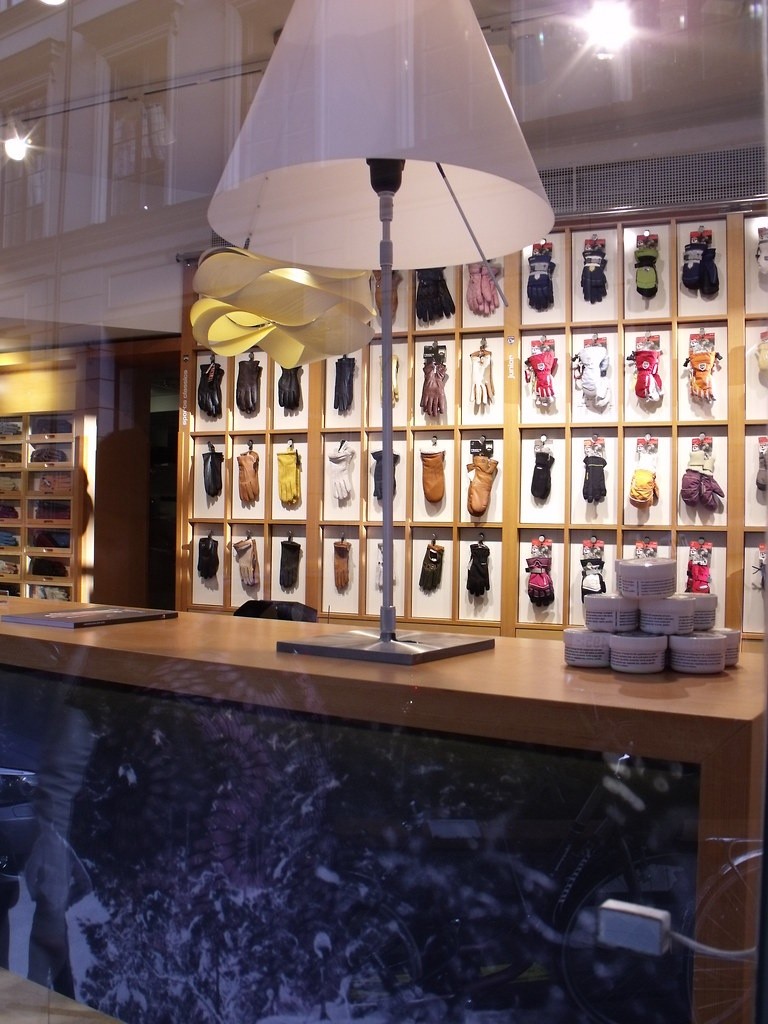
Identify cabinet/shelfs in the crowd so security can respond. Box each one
[0,410,94,603]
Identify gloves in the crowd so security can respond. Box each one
[525,555,711,608]
[197,530,300,588]
[524,344,723,408]
[527,242,719,310]
[372,262,500,323]
[755,343,768,369]
[756,451,768,491]
[333,349,494,418]
[334,537,490,597]
[328,439,498,516]
[198,358,301,417]
[200,441,303,507]
[755,240,768,275]
[530,441,725,510]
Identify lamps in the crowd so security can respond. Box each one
[3,117,29,161]
[209,0,557,667]
